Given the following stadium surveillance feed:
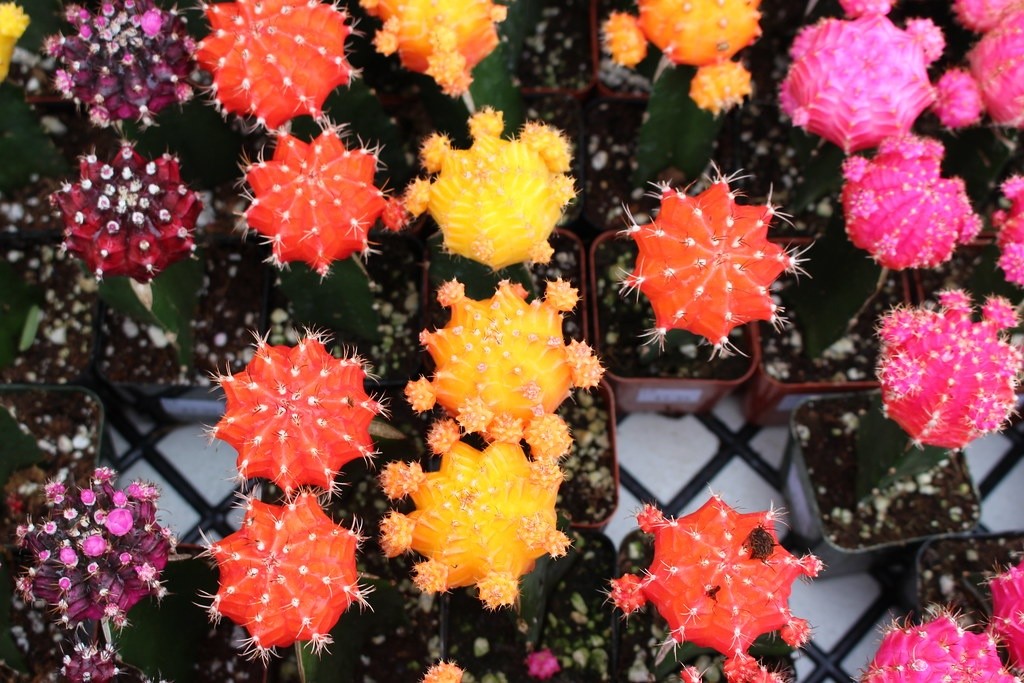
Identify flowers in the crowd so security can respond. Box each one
[0,0,1024,683]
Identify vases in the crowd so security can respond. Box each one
[180,238,270,381]
[412,230,585,352]
[262,246,416,387]
[553,379,619,542]
[97,300,183,404]
[912,238,1000,319]
[437,530,623,683]
[0,384,105,478]
[735,236,908,428]
[588,229,759,416]
[519,12,595,102]
[615,529,798,682]
[590,0,659,104]
[784,389,981,581]
[526,100,587,229]
[904,530,1023,683]
[99,543,269,683]
